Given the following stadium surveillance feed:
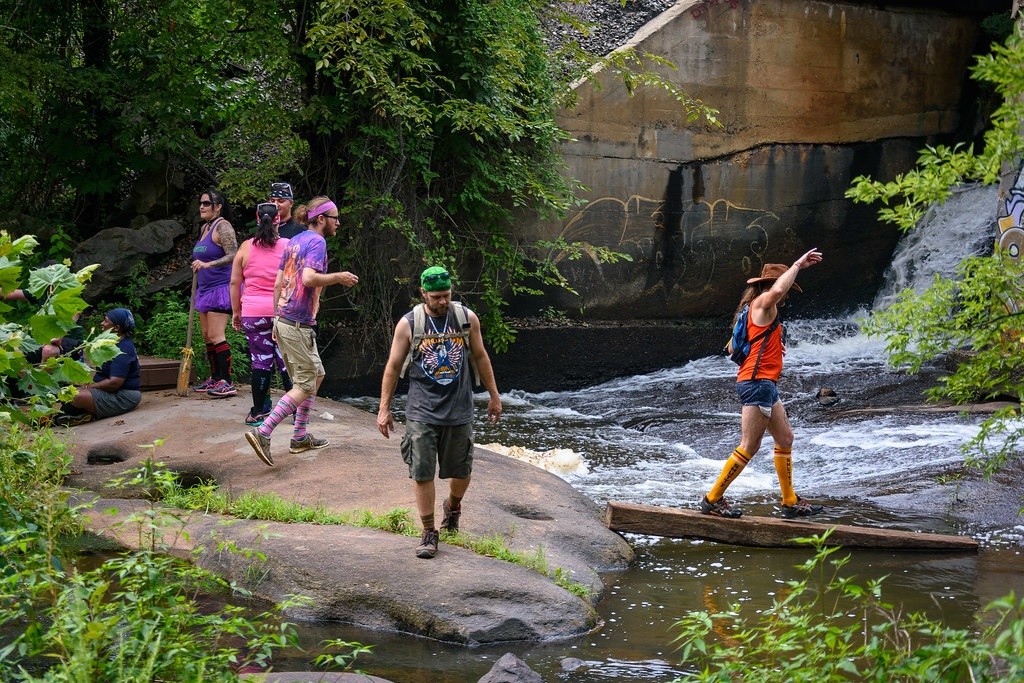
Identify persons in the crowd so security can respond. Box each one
[190,189,238,397]
[230,202,309,427]
[0,261,84,403]
[376,266,502,559]
[245,196,359,467]
[56,308,141,425]
[701,247,823,520]
[247,182,308,416]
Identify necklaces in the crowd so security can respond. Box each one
[206,215,221,231]
[426,309,450,344]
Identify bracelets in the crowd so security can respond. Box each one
[795,263,802,269]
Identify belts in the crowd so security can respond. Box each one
[278,317,310,328]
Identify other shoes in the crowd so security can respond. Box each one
[30,412,65,428]
[57,412,95,427]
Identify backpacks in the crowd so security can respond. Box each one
[722,303,780,366]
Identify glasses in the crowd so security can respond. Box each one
[257,203,277,213]
[198,201,223,207]
[423,272,450,285]
[317,214,339,223]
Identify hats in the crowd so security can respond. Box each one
[747,263,803,292]
[421,266,451,292]
[270,183,294,202]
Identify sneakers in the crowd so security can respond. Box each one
[192,376,218,392]
[440,499,461,536]
[781,494,824,519]
[701,497,743,518]
[291,410,309,425]
[245,426,274,466]
[415,529,439,557]
[208,379,237,396]
[289,433,330,453]
[262,400,272,416]
[245,407,264,426]
